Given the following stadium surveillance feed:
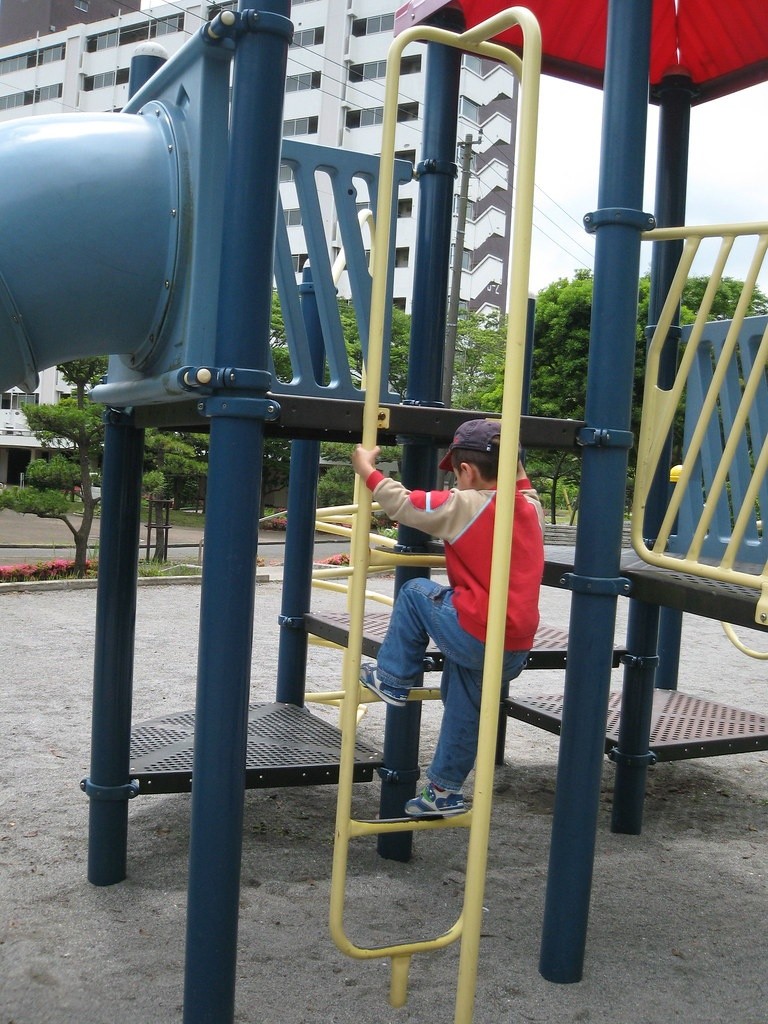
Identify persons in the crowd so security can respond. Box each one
[353,420,546,814]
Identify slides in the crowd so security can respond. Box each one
[1,108,183,394]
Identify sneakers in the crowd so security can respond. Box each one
[405,783,465,817]
[359,662,410,706]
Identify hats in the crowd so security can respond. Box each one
[439,419,520,472]
